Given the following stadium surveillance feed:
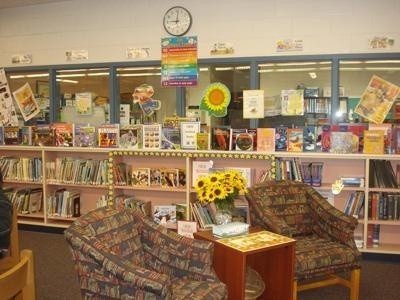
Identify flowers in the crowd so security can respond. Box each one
[194,164,251,212]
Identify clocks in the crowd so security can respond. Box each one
[163,7,192,36]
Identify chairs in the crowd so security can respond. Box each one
[0,204,35,300]
[65,182,362,300]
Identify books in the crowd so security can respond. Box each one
[341,177,365,247]
[190,199,216,228]
[1,121,400,155]
[116,194,197,237]
[97,195,107,207]
[276,157,324,186]
[112,162,186,188]
[368,159,400,247]
[46,157,109,186]
[192,161,271,186]
[2,187,43,214]
[47,188,80,217]
[0,156,42,182]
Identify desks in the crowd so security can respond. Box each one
[193,221,297,300]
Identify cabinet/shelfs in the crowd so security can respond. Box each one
[1,145,399,255]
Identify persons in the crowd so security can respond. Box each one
[0,169,13,262]
